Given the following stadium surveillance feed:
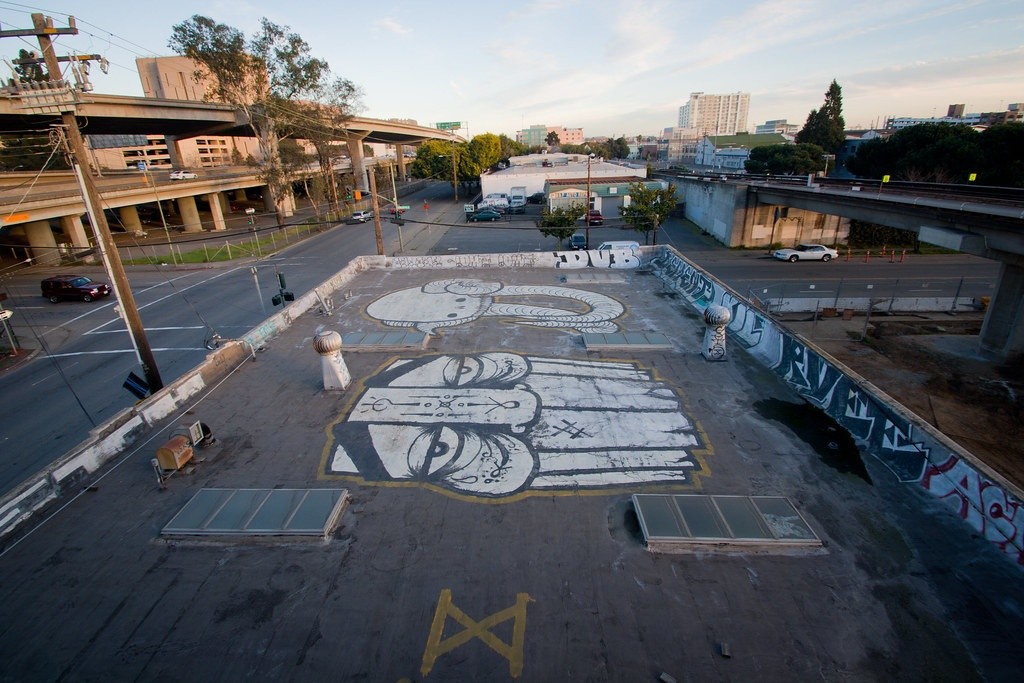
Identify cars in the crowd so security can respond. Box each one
[526,193,546,205]
[585,210,603,225]
[351,210,372,223]
[469,210,501,222]
[569,233,587,251]
[475,193,510,215]
[774,244,839,263]
[169,170,198,180]
[390,202,406,214]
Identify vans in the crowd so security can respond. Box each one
[597,241,640,251]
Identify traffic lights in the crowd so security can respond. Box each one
[345,186,352,199]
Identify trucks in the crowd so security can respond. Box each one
[509,187,527,214]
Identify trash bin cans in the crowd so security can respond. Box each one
[822,307,835,318]
[842,308,855,320]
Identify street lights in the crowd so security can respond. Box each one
[245,206,262,257]
[438,154,458,203]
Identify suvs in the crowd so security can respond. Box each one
[41,275,112,304]
